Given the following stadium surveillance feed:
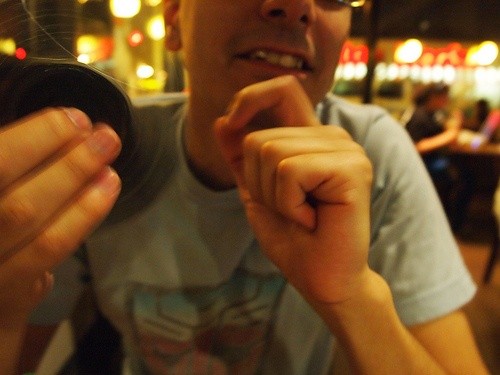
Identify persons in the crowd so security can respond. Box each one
[472,105,500,231]
[396,82,474,220]
[0,0,491,375]
[463,99,487,129]
